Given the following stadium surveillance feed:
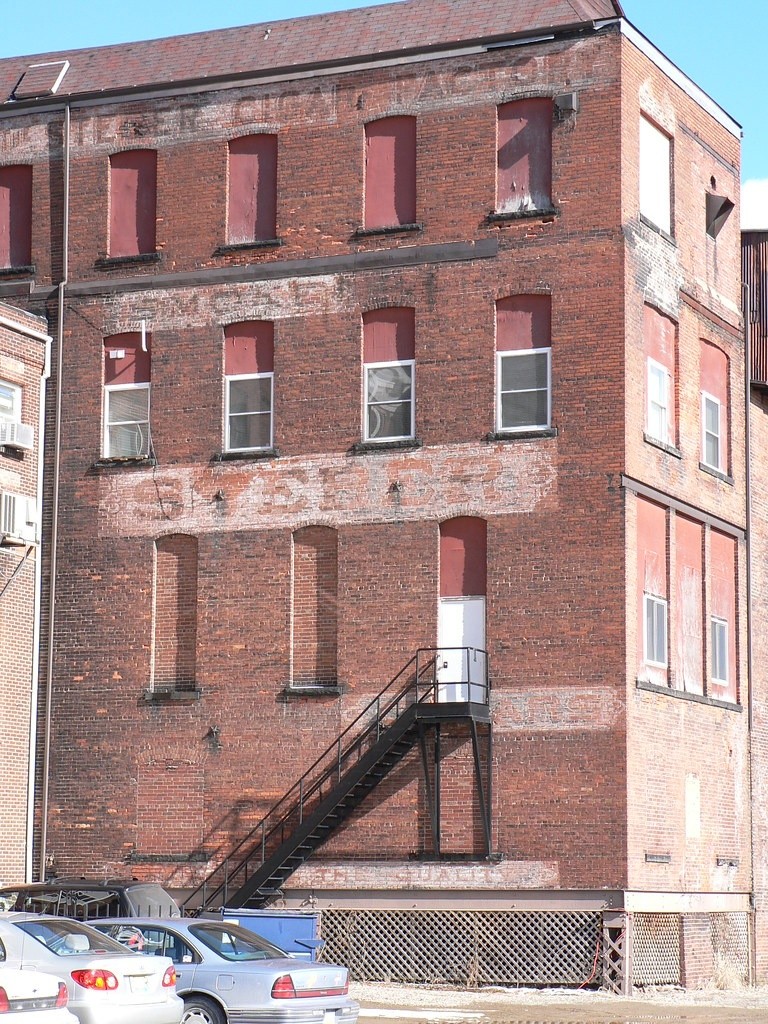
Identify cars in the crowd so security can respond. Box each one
[80,917,360,1024]
[0,911,184,1024]
[0,966,80,1024]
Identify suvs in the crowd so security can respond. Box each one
[0,876,181,919]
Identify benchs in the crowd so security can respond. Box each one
[37,933,91,954]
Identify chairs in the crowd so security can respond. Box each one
[156,948,178,961]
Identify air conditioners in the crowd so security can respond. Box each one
[0,422,35,451]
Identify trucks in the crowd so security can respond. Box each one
[195,905,322,962]
